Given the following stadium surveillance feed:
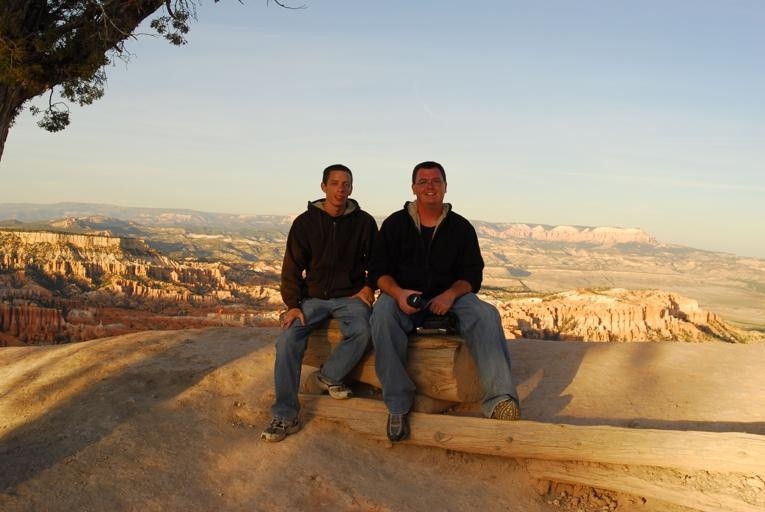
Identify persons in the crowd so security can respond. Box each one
[367,161,521,443]
[260,165,379,442]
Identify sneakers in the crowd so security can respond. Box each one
[491,398,521,420]
[314,364,352,400]
[261,415,301,441]
[386,409,410,441]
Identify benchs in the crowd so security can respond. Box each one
[303,317,485,407]
